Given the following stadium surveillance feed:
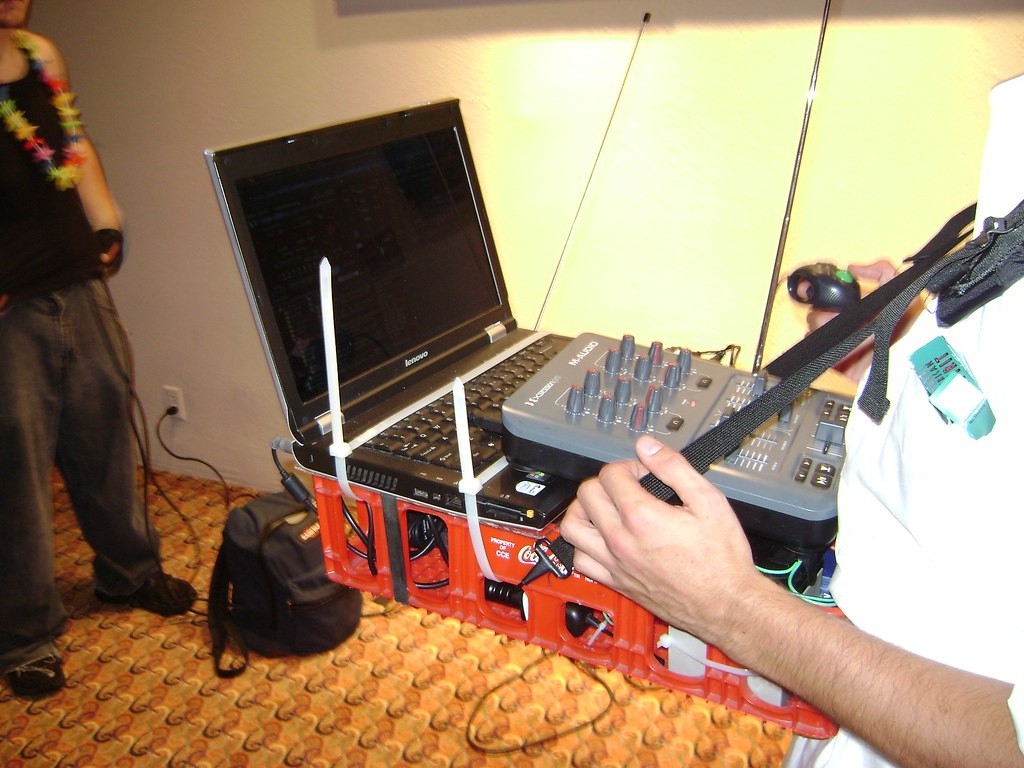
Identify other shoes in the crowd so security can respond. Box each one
[93,573,197,616]
[8,657,65,699]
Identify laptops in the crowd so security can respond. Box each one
[207,96,589,528]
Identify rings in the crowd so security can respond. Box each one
[104,265,108,276]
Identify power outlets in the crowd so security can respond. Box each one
[162,384,186,421]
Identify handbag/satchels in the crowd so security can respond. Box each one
[209,489,362,678]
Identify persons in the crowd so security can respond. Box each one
[559,74,1024,767]
[0,0,198,701]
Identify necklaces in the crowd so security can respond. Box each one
[0,31,87,191]
